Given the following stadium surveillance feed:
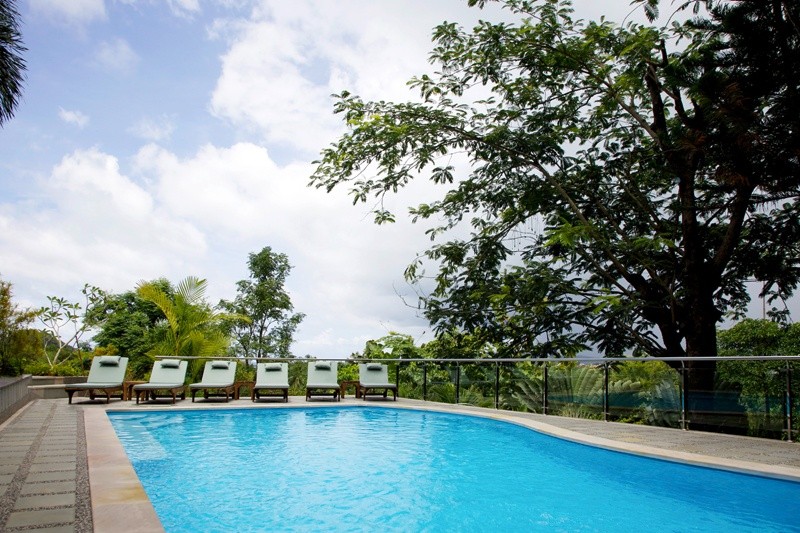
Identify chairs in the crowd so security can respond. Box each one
[305,361,340,402]
[359,362,397,402]
[254,362,290,402]
[134,359,188,405]
[65,355,129,404]
[189,360,237,402]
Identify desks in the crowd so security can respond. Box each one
[341,381,360,398]
[124,381,148,401]
[234,381,255,400]
